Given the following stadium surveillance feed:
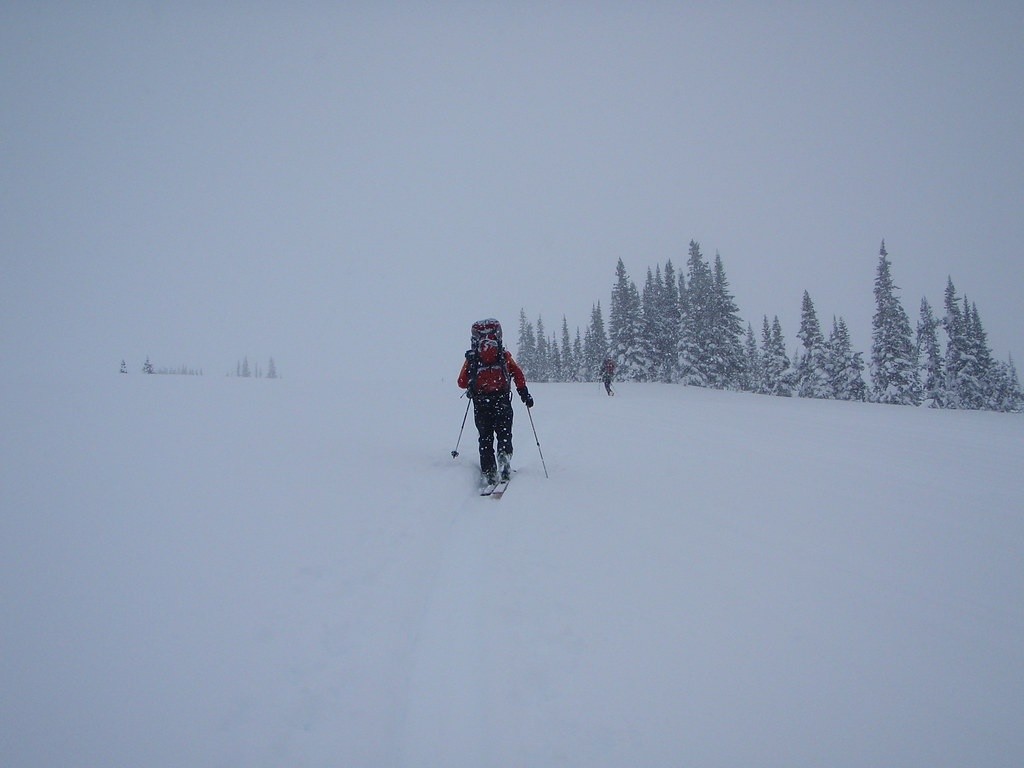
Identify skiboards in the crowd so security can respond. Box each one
[480,466,518,497]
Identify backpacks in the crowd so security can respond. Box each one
[465,318,511,389]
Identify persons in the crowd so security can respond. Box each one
[458,318,534,485]
[598,355,615,397]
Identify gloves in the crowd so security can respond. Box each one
[516,386,533,407]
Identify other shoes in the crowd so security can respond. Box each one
[502,470,509,479]
[486,468,499,485]
